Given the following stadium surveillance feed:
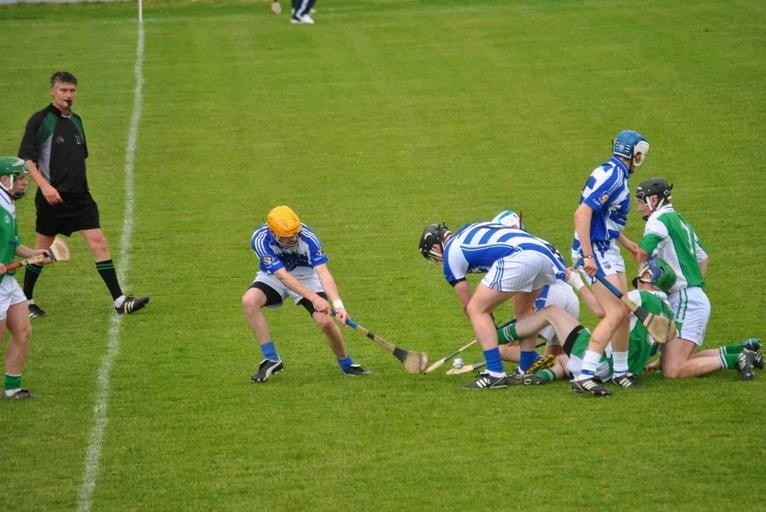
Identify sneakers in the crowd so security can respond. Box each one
[3,390,30,400]
[463,367,642,395]
[29,304,45,316]
[737,338,764,380]
[291,0,315,24]
[343,365,368,375]
[115,294,148,314]
[251,355,283,381]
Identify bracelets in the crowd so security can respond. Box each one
[581,252,595,261]
[332,299,343,310]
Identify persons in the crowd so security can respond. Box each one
[0,154,54,401]
[416,221,556,392]
[287,0,317,25]
[632,175,766,378]
[571,128,648,398]
[488,258,677,386]
[17,70,149,319]
[239,204,374,383]
[491,208,581,382]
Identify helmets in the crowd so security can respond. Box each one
[491,209,526,232]
[419,222,451,264]
[0,156,29,174]
[636,178,673,199]
[632,258,676,290]
[613,130,649,166]
[267,206,300,249]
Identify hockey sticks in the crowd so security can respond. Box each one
[328,306,428,375]
[594,271,676,345]
[424,319,516,376]
[446,341,547,376]
[1,235,72,272]
